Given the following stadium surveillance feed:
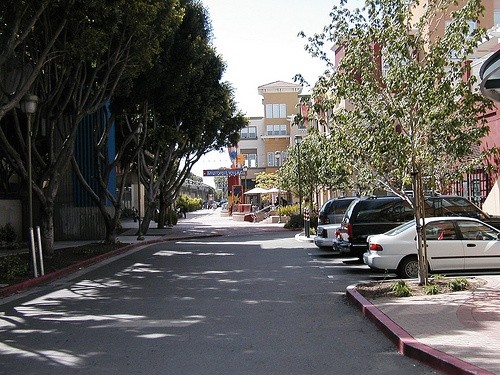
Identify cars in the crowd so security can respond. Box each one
[363,217,500,280]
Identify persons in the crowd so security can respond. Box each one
[131,206,138,222]
[280,197,288,208]
[273,197,281,208]
[261,203,264,209]
[228,205,233,216]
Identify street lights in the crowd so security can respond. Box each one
[19,93,40,273]
[294,135,303,216]
[242,165,248,204]
[134,122,145,241]
[274,151,282,223]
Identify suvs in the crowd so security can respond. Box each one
[332,191,500,259]
[313,196,368,249]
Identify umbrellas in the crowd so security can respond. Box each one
[268,186,288,205]
[243,187,268,205]
